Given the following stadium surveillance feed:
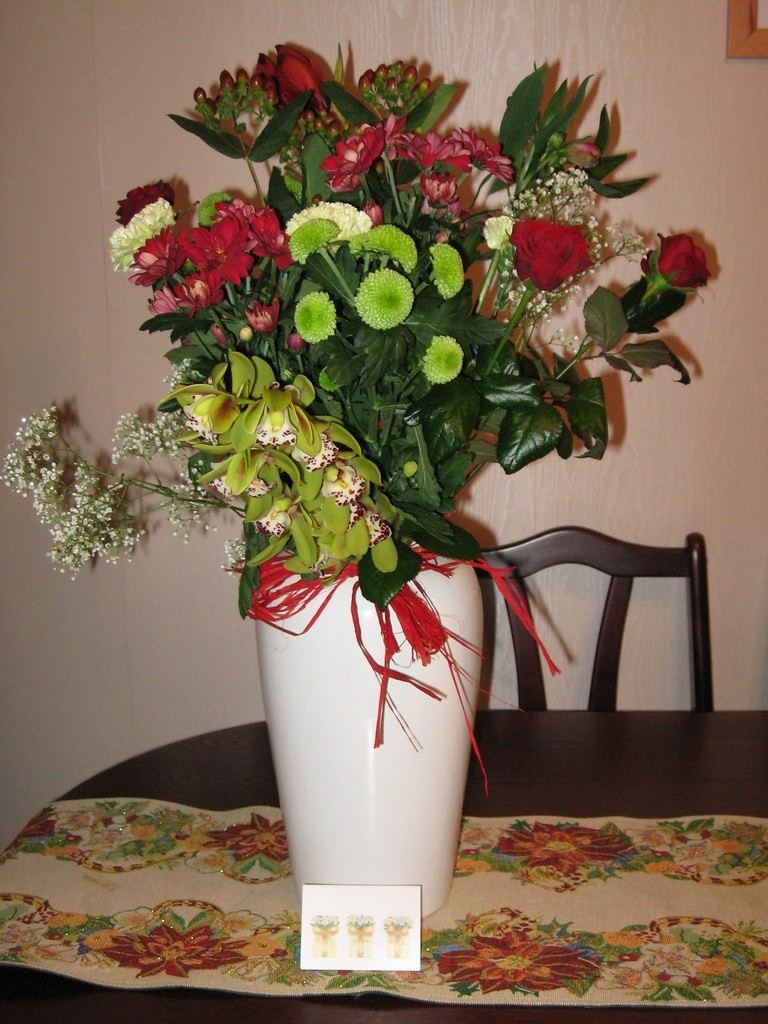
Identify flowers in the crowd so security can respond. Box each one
[1,40,714,618]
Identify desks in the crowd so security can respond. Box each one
[0,709,768,1024]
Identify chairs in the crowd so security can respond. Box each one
[468,528,716,711]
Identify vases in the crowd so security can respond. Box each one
[246,542,481,920]
